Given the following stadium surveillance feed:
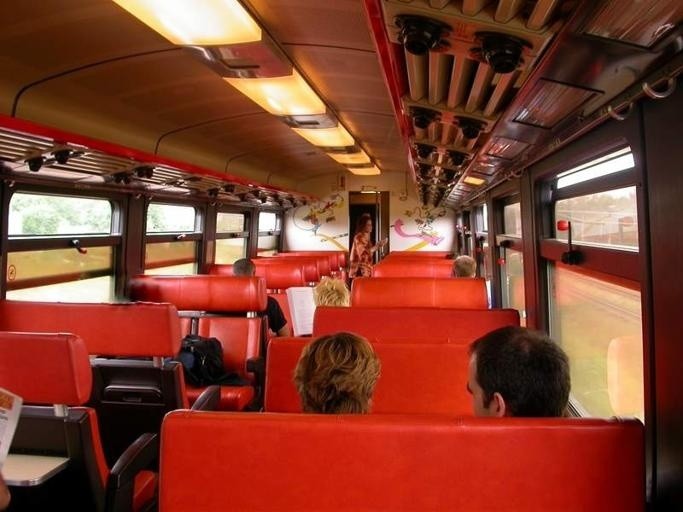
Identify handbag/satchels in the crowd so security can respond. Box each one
[176,333,225,389]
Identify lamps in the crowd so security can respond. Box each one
[113,0,381,176]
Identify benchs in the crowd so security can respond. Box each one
[0,251,646,511]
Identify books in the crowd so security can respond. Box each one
[282,284,316,336]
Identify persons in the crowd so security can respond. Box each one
[230,257,289,336]
[314,276,351,307]
[444,252,459,259]
[465,324,571,418]
[450,255,478,277]
[0,473,11,511]
[348,214,388,289]
[291,329,382,415]
